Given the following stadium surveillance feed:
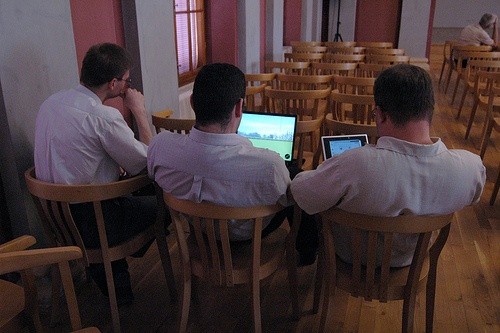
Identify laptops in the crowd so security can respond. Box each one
[236,111,297,174]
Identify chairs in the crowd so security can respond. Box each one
[438,40,500,205]
[0,41,454,333]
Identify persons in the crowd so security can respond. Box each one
[33,42,172,240]
[451,12,500,68]
[290,63,486,271]
[146,63,292,244]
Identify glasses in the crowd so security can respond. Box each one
[370,108,377,122]
[117,78,132,85]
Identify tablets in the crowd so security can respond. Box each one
[321,133,369,160]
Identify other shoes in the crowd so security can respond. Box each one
[298,253,316,265]
[117,294,133,305]
